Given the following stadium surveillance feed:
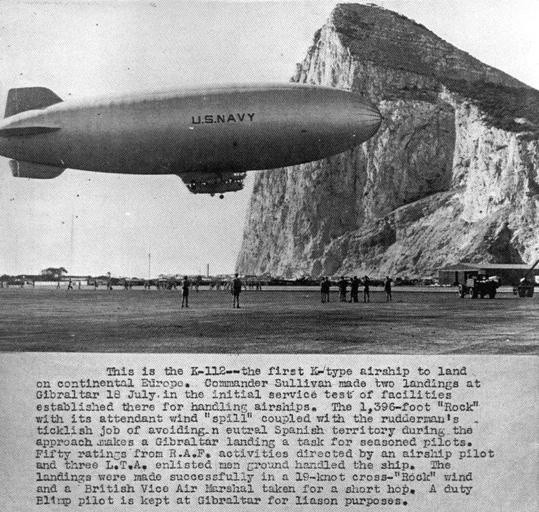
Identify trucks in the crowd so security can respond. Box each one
[458,271,499,299]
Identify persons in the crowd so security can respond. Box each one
[231,273,242,308]
[180,274,190,308]
[106,280,112,296]
[319,274,371,303]
[124,279,262,293]
[383,276,393,303]
